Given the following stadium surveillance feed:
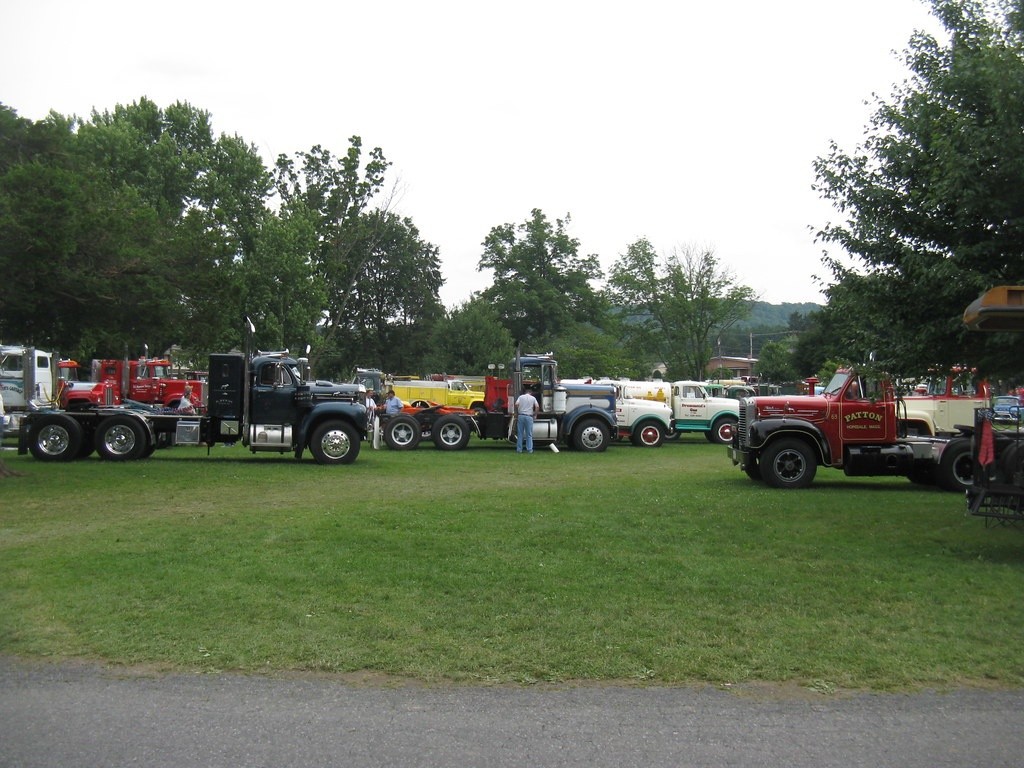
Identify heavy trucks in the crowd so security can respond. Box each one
[727,367,1024,490]
[19,317,371,469]
[381,351,622,453]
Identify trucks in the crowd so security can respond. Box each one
[350,365,488,411]
[0,341,208,422]
[562,371,756,446]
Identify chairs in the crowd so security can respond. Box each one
[965,469,1024,528]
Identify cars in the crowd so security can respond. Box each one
[994,395,1021,423]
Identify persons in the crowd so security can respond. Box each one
[0,383,6,438]
[365,389,404,423]
[178,386,196,414]
[515,386,540,454]
